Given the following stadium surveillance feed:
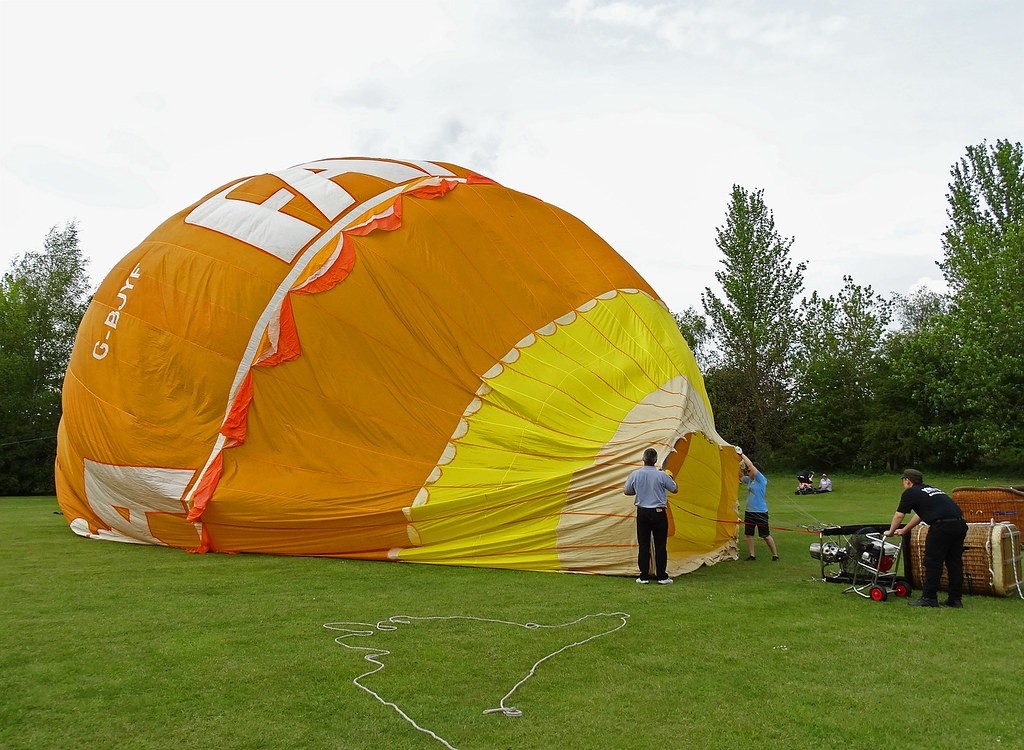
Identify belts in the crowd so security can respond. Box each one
[933,516,963,523]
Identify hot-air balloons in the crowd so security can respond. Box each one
[51,158,1021,599]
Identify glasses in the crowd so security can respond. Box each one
[746,470,751,472]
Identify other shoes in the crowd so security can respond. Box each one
[772,555,779,561]
[939,598,964,608]
[636,577,650,584]
[658,577,673,584]
[744,556,757,560]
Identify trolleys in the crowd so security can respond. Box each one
[843,531,913,601]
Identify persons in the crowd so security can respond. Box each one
[735,445,779,561]
[884,469,969,609]
[814,474,832,494]
[624,448,678,584]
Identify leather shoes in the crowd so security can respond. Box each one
[907,596,939,607]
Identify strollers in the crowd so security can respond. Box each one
[794,470,816,495]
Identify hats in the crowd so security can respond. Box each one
[822,474,827,476]
[900,469,923,483]
